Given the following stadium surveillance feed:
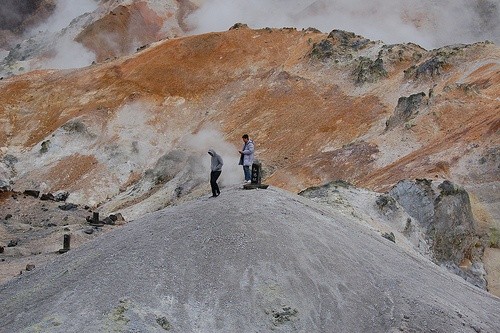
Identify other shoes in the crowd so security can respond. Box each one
[243,180,251,185]
[210,192,220,197]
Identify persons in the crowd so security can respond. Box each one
[238,134,254,183]
[208,148,223,197]
